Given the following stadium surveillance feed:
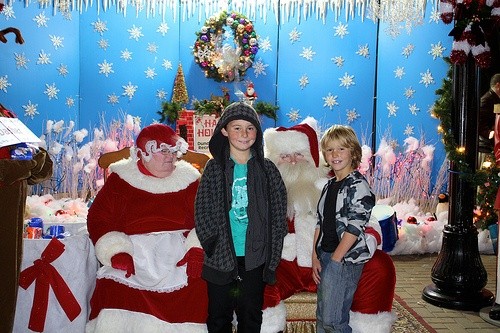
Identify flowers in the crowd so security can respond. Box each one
[192,10,259,83]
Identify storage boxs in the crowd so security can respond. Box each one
[12,234,100,333]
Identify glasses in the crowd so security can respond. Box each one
[160,149,178,157]
[280,153,305,162]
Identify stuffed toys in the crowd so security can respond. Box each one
[244,83,257,104]
[0,105,54,333]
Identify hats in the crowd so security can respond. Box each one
[263,116,321,170]
[130,123,188,162]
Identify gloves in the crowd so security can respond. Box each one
[111,251,136,278]
[176,247,205,282]
[366,233,377,258]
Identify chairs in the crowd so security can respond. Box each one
[97,147,211,186]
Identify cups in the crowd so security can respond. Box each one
[29,218,42,227]
[47,225,64,238]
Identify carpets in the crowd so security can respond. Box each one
[387,294,437,333]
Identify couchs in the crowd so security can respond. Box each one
[232,290,317,333]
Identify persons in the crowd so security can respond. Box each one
[232,123,398,333]
[195,102,288,333]
[311,124,376,333]
[85,125,209,333]
[473,74,500,232]
